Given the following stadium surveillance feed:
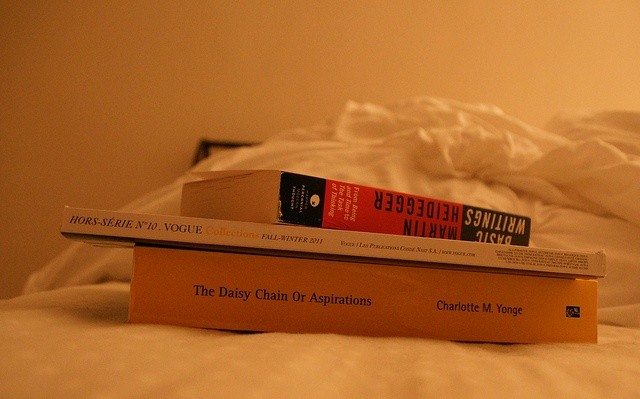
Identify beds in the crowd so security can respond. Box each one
[0,136,640,399]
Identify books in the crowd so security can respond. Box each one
[178,169,532,247]
[129,244,598,346]
[59,204,608,280]
